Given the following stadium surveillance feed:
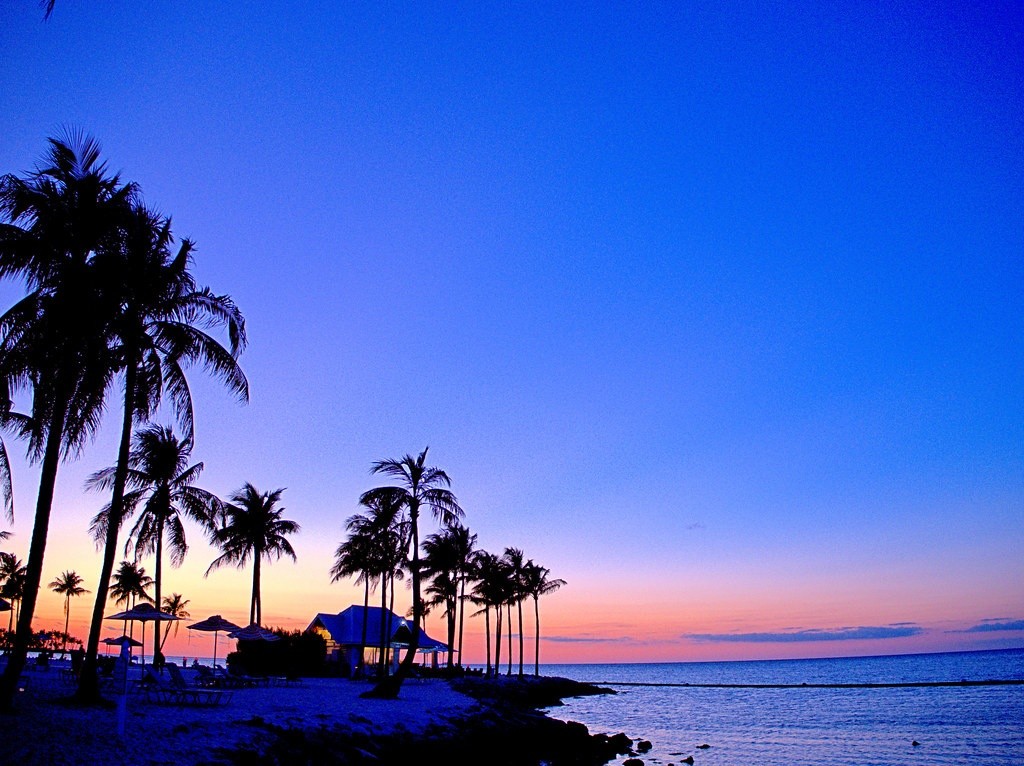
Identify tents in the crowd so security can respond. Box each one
[417,643,459,671]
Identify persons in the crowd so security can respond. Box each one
[158,648,165,675]
[450,663,462,671]
[193,657,199,667]
[79,645,85,660]
[182,657,187,668]
[466,666,483,677]
[422,663,425,670]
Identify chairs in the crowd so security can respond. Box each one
[20,652,288,707]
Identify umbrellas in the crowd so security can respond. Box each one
[103,603,184,665]
[97,636,143,666]
[227,622,281,676]
[187,616,242,682]
